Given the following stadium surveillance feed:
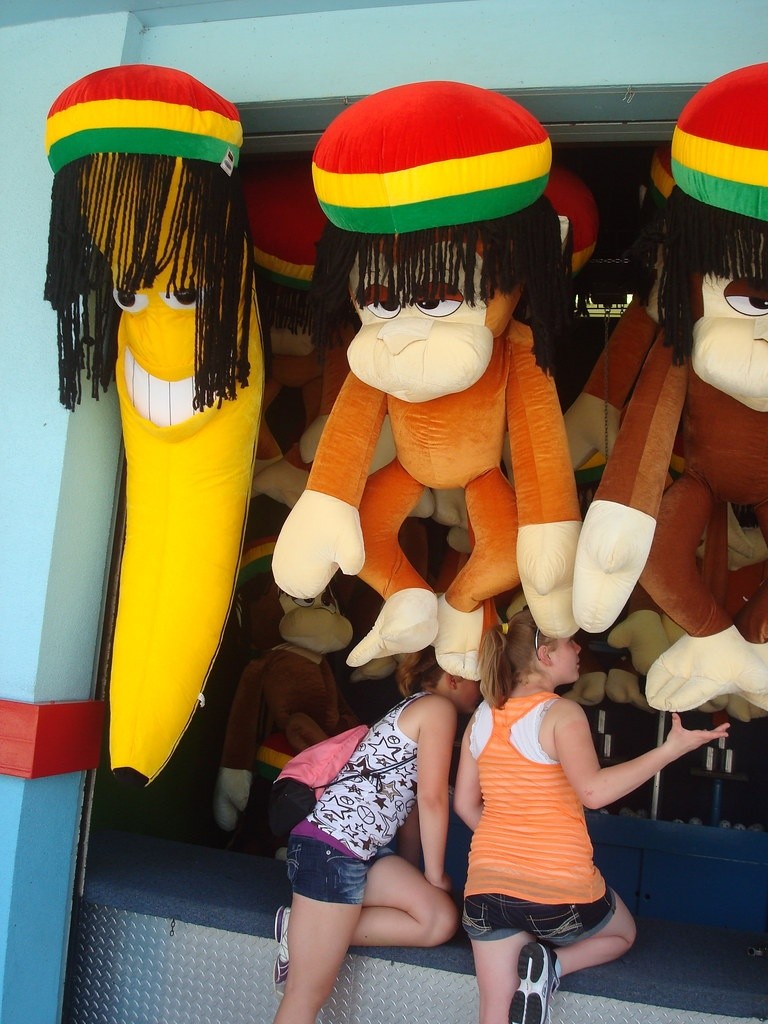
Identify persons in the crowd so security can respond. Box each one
[453,609,730,1024]
[274,641,481,1024]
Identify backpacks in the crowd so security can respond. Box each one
[268,695,420,837]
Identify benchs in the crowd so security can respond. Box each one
[64,825,768,1024]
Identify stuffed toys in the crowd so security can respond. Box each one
[45,60,768,833]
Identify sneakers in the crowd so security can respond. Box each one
[273,905,292,984]
[509,941,561,1024]
